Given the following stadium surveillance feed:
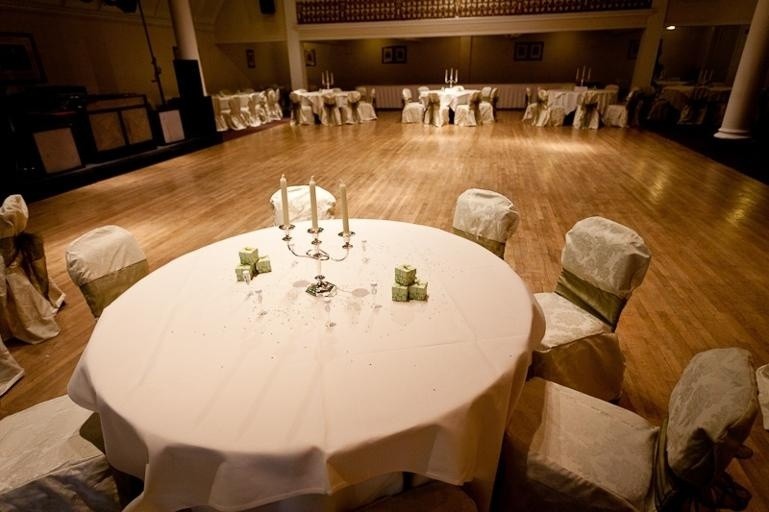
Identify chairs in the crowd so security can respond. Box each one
[64,223,152,324]
[491,347,759,512]
[449,187,520,259]
[212,88,283,130]
[531,214,653,403]
[0,189,64,344]
[401,85,497,127]
[289,88,377,126]
[523,86,707,128]
[270,184,337,228]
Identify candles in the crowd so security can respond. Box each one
[321,72,324,84]
[326,71,329,83]
[280,178,289,224]
[450,68,453,79]
[576,67,579,79]
[340,185,350,234]
[588,68,591,80]
[445,69,448,83]
[308,181,318,228]
[331,72,333,83]
[455,70,458,81]
[581,66,584,79]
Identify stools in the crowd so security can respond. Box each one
[0,394,120,512]
[0,333,29,397]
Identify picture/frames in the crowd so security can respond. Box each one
[246,49,256,69]
[514,42,544,60]
[304,49,316,67]
[381,46,407,64]
